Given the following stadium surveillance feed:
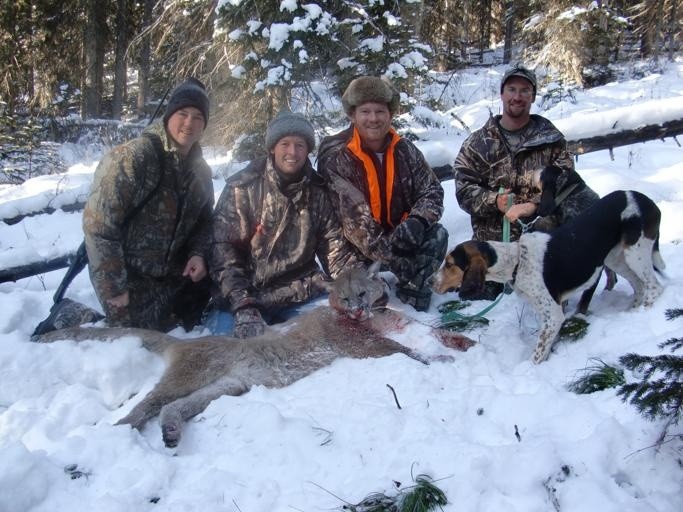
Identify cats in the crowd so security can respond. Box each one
[27,254,478,449]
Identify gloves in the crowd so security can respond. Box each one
[235,307,271,341]
[384,217,433,284]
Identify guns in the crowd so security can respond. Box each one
[53,241,88,303]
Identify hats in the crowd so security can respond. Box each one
[342,76,400,119]
[163,76,210,132]
[265,111,316,153]
[501,61,537,94]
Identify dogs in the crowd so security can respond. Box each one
[421,190,665,365]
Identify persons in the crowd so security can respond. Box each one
[453,65,574,301]
[30,77,217,338]
[313,76,449,311]
[194,103,367,342]
